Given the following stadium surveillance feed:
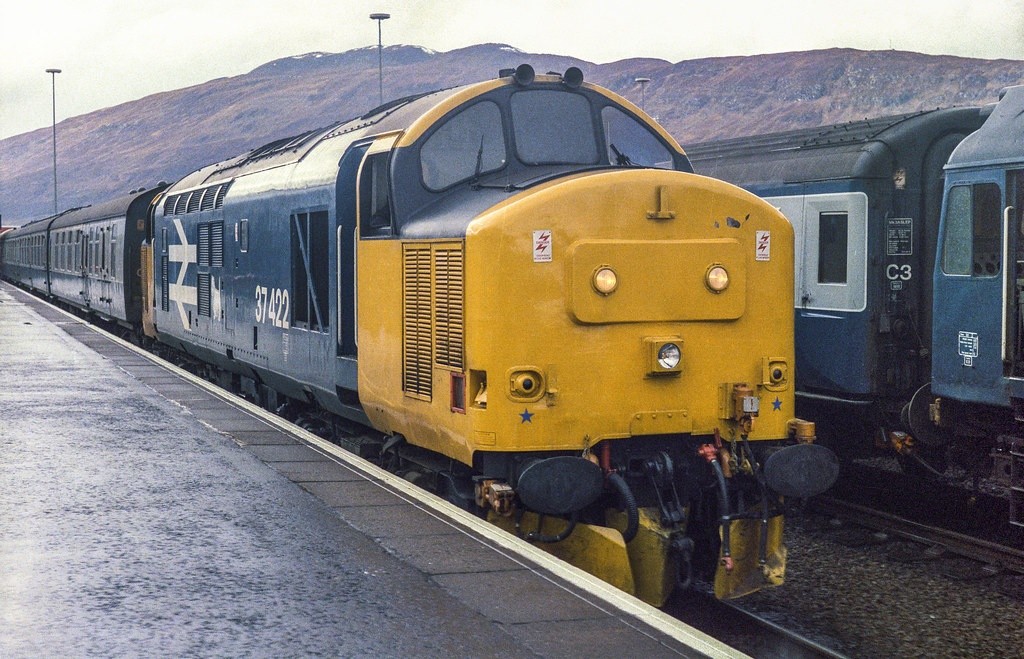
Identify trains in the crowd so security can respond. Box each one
[680,85,1023,530]
[0,64,842,611]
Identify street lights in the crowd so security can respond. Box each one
[634,78,650,111]
[369,13,391,107]
[45,69,62,216]
